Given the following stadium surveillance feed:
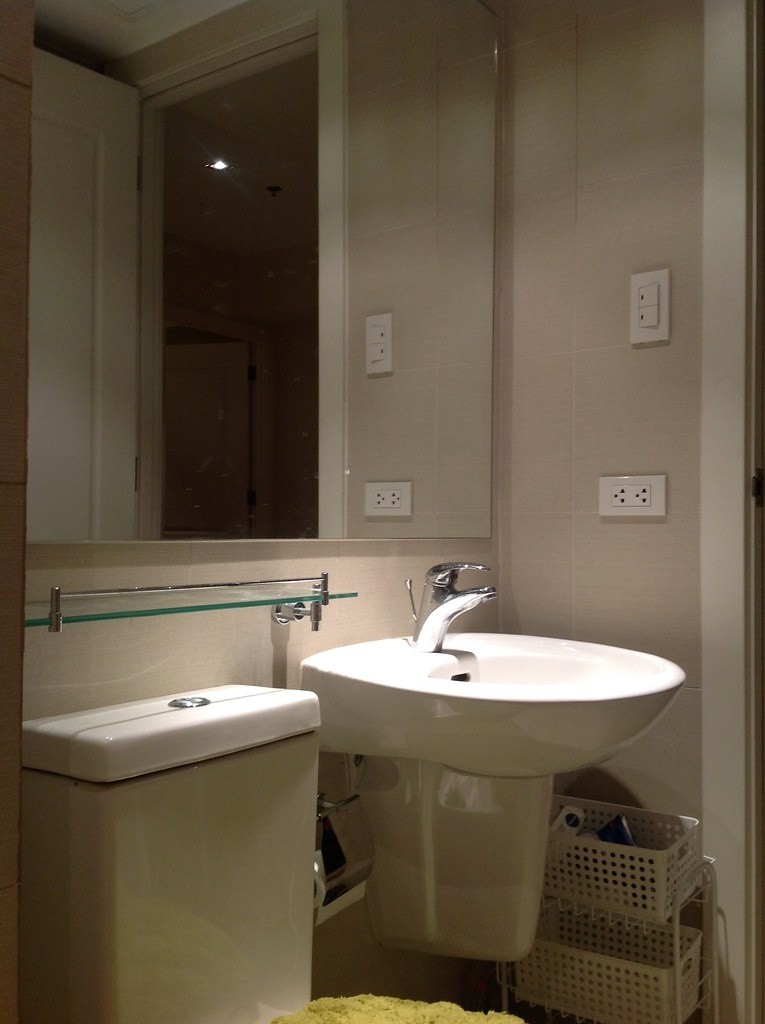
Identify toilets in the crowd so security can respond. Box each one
[18,680,530,1024]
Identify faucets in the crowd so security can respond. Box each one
[411,560,497,652]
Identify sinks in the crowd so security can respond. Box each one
[298,631,686,778]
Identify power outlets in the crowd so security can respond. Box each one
[599,475,666,517]
[365,482,411,515]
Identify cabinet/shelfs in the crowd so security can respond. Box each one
[497,793,719,1024]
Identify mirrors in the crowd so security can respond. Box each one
[26,0,501,544]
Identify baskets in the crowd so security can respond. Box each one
[517,899,702,1024]
[544,794,703,922]
